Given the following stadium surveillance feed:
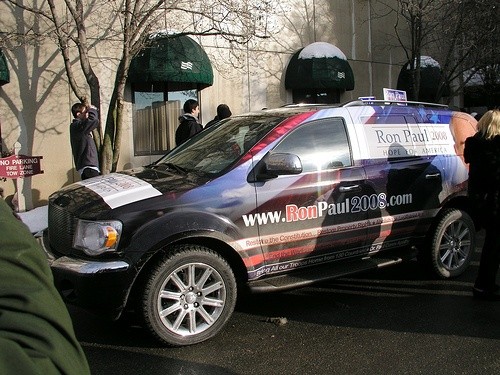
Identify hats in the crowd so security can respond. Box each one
[217,104,232,118]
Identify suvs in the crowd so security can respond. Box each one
[33,99,484,346]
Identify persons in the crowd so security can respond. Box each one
[69,96,101,180]
[463,109,500,302]
[175,99,203,146]
[0,196,91,375]
[203,104,232,130]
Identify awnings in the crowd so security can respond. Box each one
[285,45,355,91]
[397,57,450,97]
[128,35,214,89]
